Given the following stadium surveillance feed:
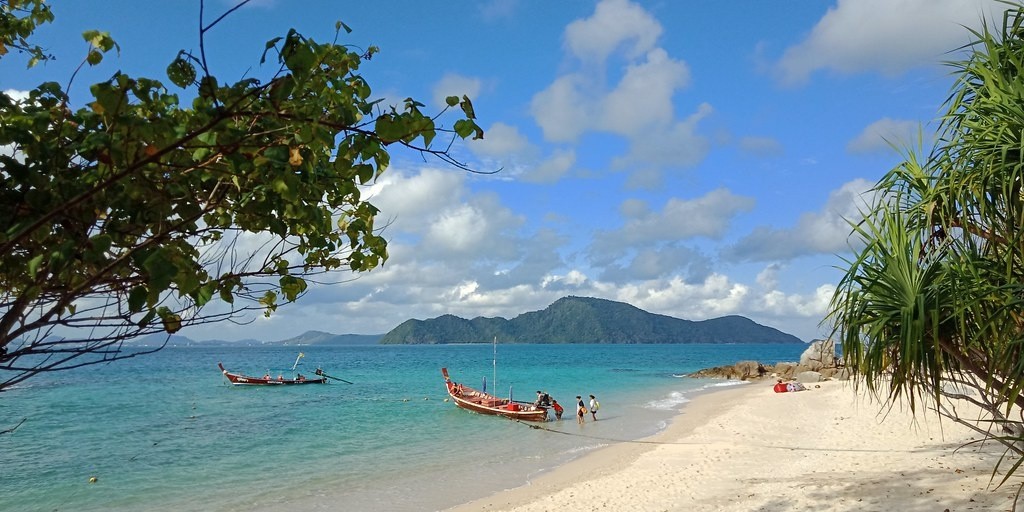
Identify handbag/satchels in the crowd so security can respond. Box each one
[582,408,588,414]
[596,402,600,409]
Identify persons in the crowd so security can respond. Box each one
[264,373,300,381]
[450,382,598,424]
[758,364,765,377]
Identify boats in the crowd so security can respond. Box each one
[773,381,789,393]
[441,336,547,423]
[218,352,353,385]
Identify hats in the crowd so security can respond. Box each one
[576,395,581,398]
